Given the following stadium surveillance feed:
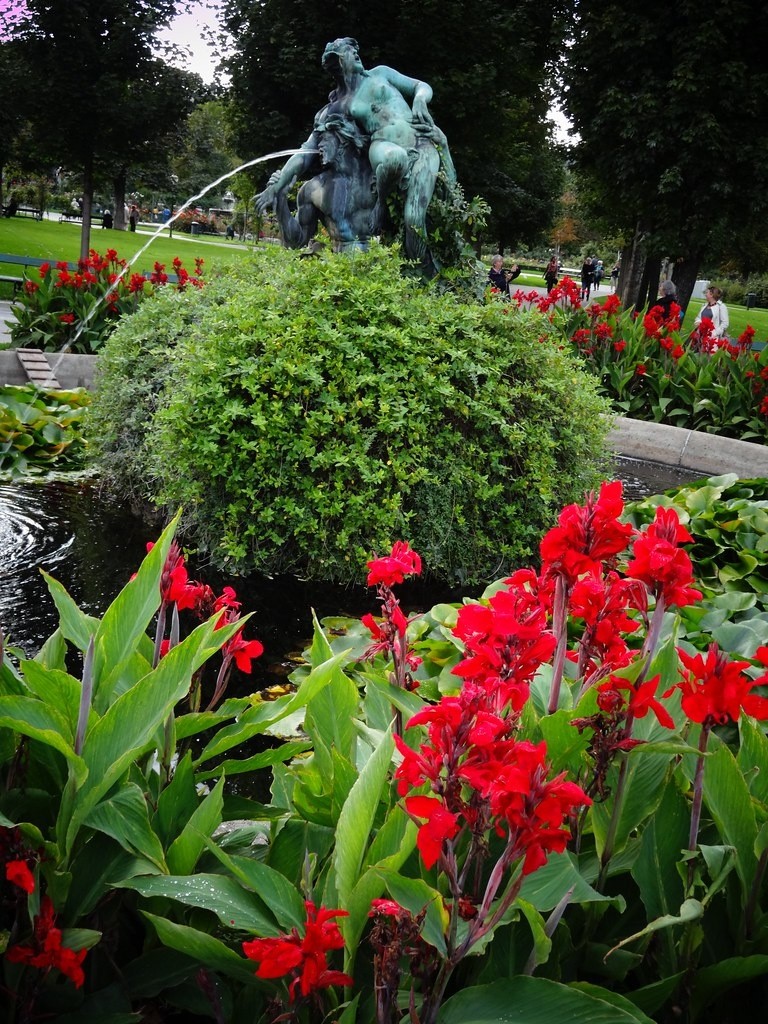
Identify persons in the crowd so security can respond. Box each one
[581,258,622,302]
[653,279,678,321]
[488,254,521,302]
[69,197,171,233]
[542,256,558,293]
[251,37,456,271]
[694,287,729,356]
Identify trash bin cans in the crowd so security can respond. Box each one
[191,222,199,234]
[747,294,756,308]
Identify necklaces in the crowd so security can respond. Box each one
[708,301,717,306]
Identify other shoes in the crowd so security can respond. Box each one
[553,278,558,284]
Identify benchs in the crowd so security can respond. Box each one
[728,338,767,350]
[14,208,41,219]
[60,212,105,229]
